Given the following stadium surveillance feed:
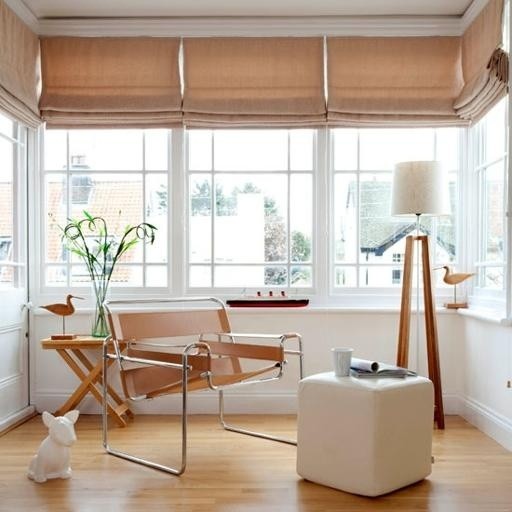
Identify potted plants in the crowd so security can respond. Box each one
[54,208,158,337]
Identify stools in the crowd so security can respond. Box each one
[292,371,436,498]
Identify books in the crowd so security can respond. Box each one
[350,355,417,379]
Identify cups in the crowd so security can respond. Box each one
[332,348,354,376]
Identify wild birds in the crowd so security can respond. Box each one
[431,266,477,304]
[38,294,86,335]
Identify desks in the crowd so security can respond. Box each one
[40,335,135,427]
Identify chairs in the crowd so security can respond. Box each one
[102,295,304,476]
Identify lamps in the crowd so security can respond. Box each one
[391,160,452,428]
